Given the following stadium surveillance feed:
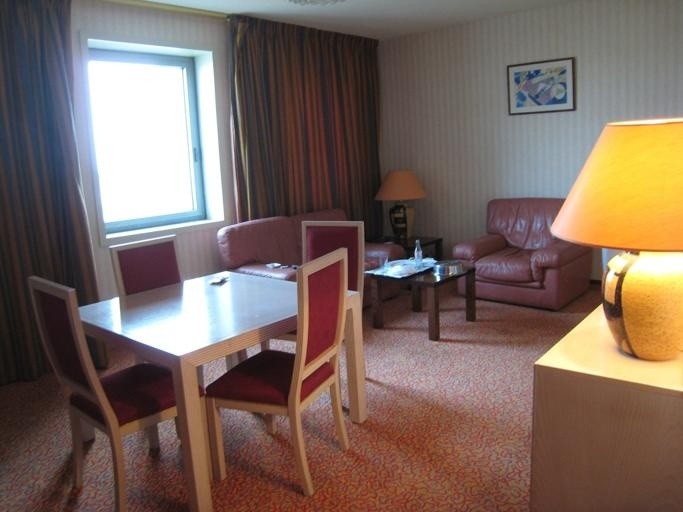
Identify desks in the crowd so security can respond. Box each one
[78,272,368,512]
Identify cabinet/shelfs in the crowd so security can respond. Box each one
[530,303,683,512]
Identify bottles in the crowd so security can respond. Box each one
[414,240,422,270]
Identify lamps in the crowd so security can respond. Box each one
[374,170,426,239]
[550,117,683,363]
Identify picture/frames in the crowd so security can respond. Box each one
[506,58,575,115]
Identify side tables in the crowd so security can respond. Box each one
[381,235,445,261]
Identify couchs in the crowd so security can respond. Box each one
[452,197,592,311]
[219,205,403,335]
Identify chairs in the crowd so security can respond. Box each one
[31,274,206,512]
[110,234,236,392]
[204,249,348,496]
[261,221,363,355]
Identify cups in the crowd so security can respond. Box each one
[378,255,389,273]
